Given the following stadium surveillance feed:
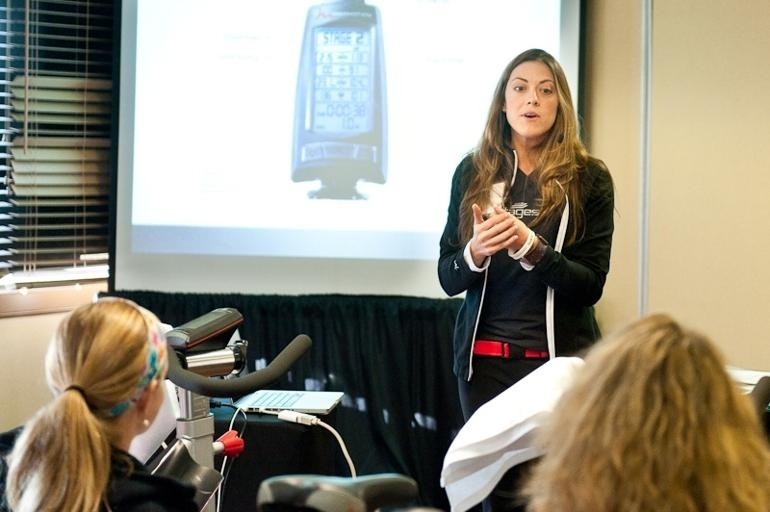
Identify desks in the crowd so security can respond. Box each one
[212,396,346,512]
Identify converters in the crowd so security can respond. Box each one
[277,410,320,426]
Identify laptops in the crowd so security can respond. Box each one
[225,327,345,415]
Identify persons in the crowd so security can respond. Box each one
[436,48,614,511]
[515,313,768,512]
[0,298,197,512]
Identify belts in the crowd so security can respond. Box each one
[473,339,548,360]
